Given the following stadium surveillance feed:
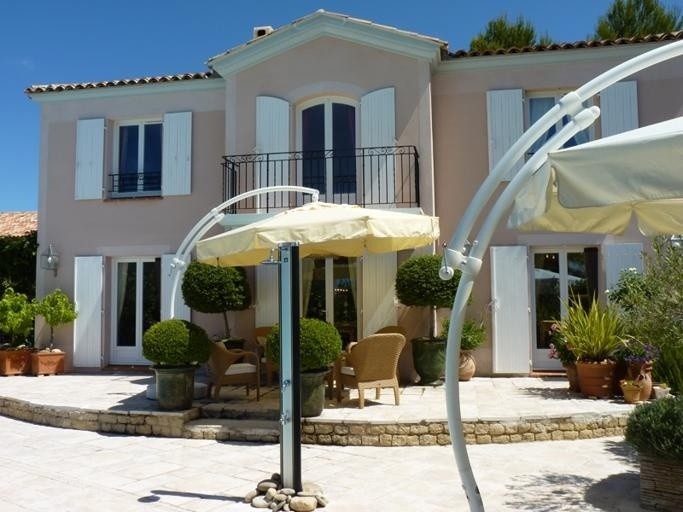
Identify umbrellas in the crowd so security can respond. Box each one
[437,35,682,512]
[314,262,355,281]
[165,185,441,321]
[534,267,585,295]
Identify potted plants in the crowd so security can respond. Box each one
[266,317,341,418]
[0,281,73,378]
[394,252,488,387]
[545,283,682,512]
[142,259,251,411]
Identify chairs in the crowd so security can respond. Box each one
[206,327,274,404]
[326,325,409,411]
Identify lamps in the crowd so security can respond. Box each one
[38,241,60,278]
